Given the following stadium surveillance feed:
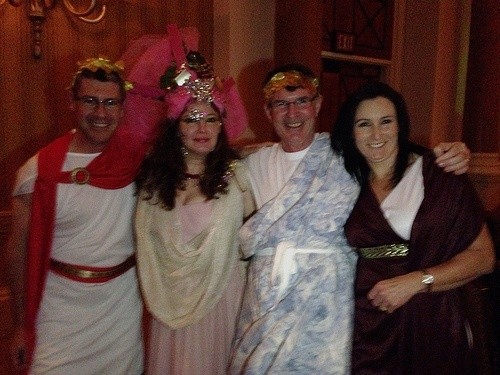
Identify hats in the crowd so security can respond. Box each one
[159,50,250,143]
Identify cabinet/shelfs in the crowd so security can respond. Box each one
[273,0,406,135]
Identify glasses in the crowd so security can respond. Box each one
[76,96,121,109]
[269,95,318,111]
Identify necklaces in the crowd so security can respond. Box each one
[182,170,206,190]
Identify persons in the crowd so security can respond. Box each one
[245,64,472,374]
[4,57,147,375]
[133,51,257,375]
[330,81,495,375]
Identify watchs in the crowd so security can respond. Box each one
[421,268,434,294]
